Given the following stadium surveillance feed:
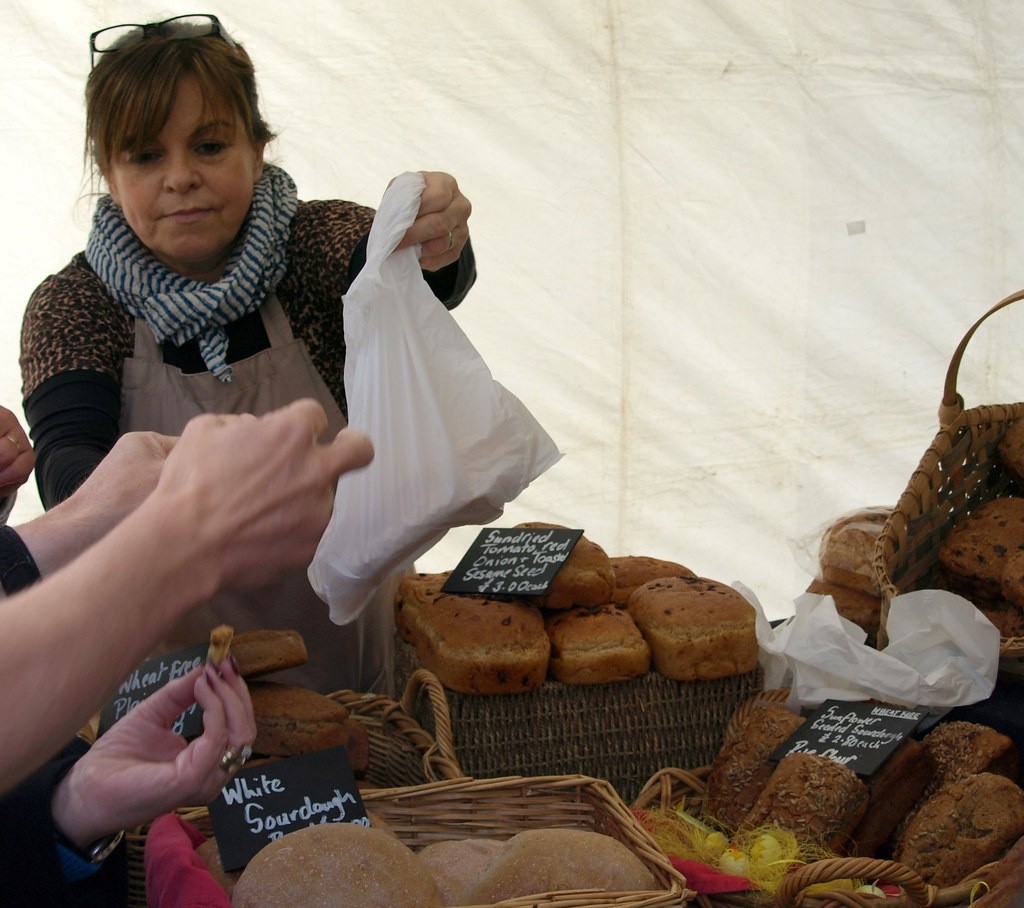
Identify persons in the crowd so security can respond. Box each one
[18,22,477,694]
[0,406,376,908]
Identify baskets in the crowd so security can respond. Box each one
[75,668,467,908]
[631,764,1024,908]
[388,617,766,812]
[869,288,1024,673]
[157,774,697,908]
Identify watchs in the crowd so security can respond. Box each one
[89,830,125,863]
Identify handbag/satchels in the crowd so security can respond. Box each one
[306,172,566,626]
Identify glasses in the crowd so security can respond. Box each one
[87,13,238,71]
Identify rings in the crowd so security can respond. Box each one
[7,435,22,450]
[219,747,246,774]
[449,232,454,250]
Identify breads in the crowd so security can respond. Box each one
[390,519,757,694]
[222,627,377,791]
[703,417,1024,891]
[193,807,658,908]
